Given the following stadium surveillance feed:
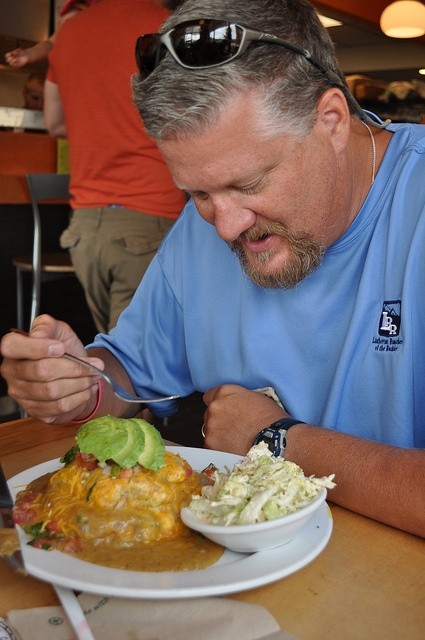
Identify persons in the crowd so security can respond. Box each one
[6,1,90,69]
[0,0,425,534]
[46,1,197,345]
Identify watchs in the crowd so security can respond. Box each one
[250,415,304,462]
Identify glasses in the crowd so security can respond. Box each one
[135,18,392,130]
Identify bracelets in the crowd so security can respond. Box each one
[70,380,104,426]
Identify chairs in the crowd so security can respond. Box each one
[9,173,75,330]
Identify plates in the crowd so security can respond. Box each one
[4,446,334,603]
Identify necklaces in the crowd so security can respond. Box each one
[351,111,385,188]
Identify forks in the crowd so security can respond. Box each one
[10,327,181,404]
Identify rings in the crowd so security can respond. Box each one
[200,420,209,439]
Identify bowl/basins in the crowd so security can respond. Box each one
[179,476,329,554]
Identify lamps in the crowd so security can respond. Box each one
[380,0,425,38]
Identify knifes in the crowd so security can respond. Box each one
[0,467,26,576]
